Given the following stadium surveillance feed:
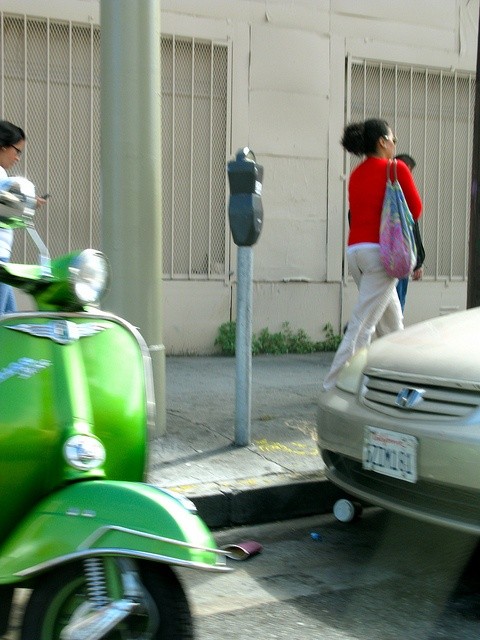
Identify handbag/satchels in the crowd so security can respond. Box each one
[380,159,425,278]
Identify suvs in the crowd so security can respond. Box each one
[0,173,236,640]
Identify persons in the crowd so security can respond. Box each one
[323,119,423,393]
[0,121,48,314]
[342,154,425,338]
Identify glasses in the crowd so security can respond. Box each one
[8,138,18,152]
[384,135,398,144]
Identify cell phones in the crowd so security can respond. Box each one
[40,193,52,200]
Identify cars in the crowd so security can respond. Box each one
[318,307,479,537]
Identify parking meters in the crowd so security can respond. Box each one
[226,147,263,446]
